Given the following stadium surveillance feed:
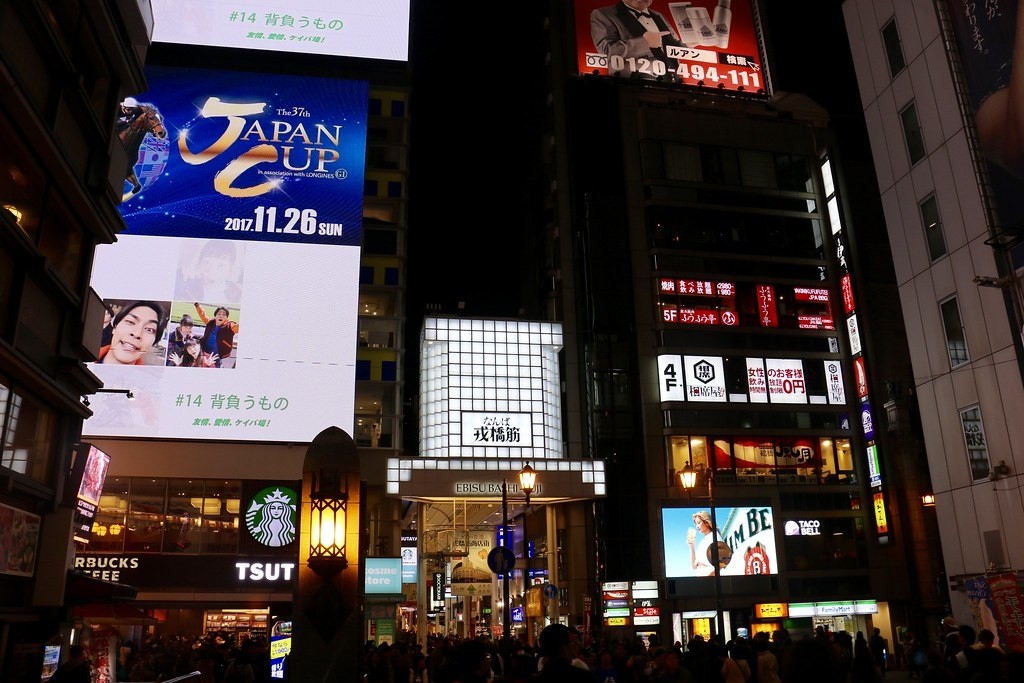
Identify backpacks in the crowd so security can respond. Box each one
[227,663,248,683]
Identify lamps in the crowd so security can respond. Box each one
[308,467,348,579]
[82,395,90,406]
[918,490,935,511]
[127,391,133,398]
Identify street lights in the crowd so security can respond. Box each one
[677,460,727,648]
[515,460,538,653]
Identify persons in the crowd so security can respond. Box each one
[48,632,270,683]
[688,510,725,576]
[592,0,683,82]
[94,302,239,369]
[361,618,1024,683]
